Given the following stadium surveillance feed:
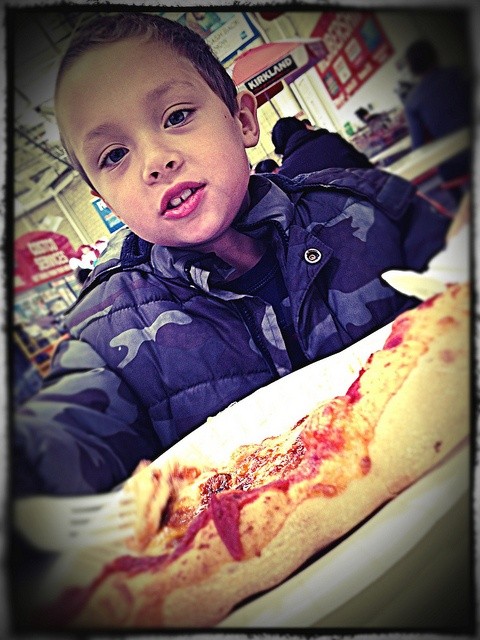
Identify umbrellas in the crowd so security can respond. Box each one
[223,38,329,119]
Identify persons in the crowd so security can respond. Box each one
[354,104,390,125]
[254,156,279,175]
[269,113,371,176]
[10,9,455,499]
[401,44,448,149]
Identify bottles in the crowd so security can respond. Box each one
[343,121,354,136]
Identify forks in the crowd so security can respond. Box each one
[16,490,139,554]
